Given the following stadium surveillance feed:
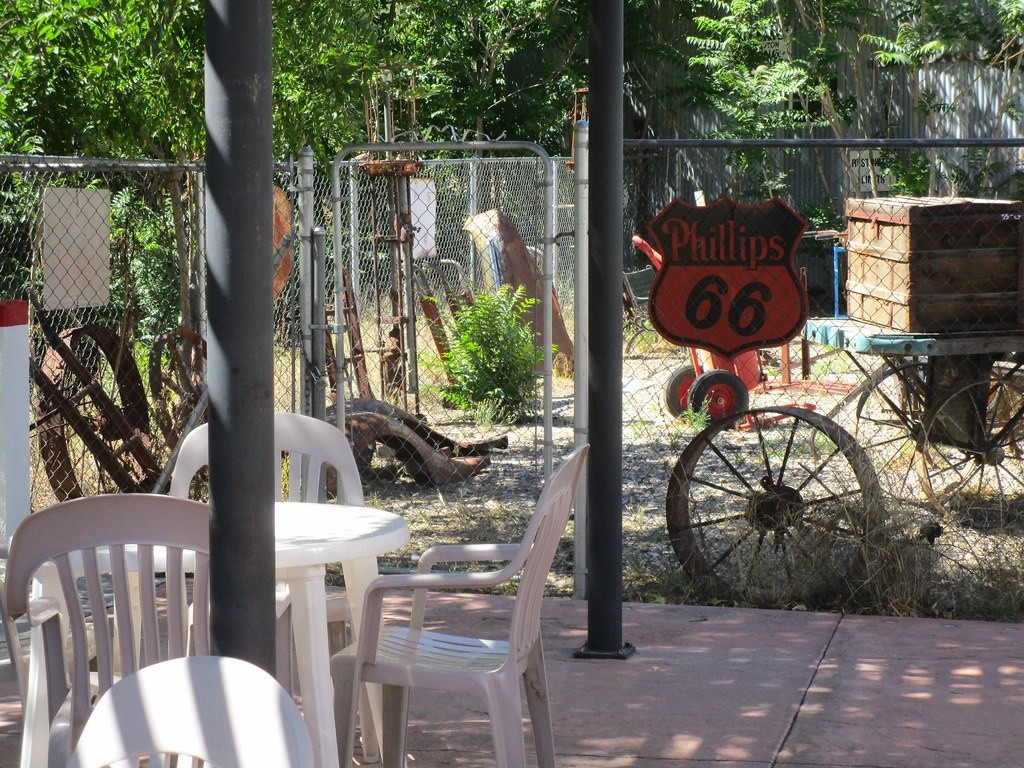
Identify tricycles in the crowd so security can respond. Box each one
[801,317,1024,530]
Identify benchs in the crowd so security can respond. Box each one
[622,265,657,356]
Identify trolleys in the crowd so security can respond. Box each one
[631,235,816,430]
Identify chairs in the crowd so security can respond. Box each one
[63,655,316,768]
[5,493,211,760]
[169,411,366,506]
[329,444,590,768]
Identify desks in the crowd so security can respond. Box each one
[5,501,412,768]
